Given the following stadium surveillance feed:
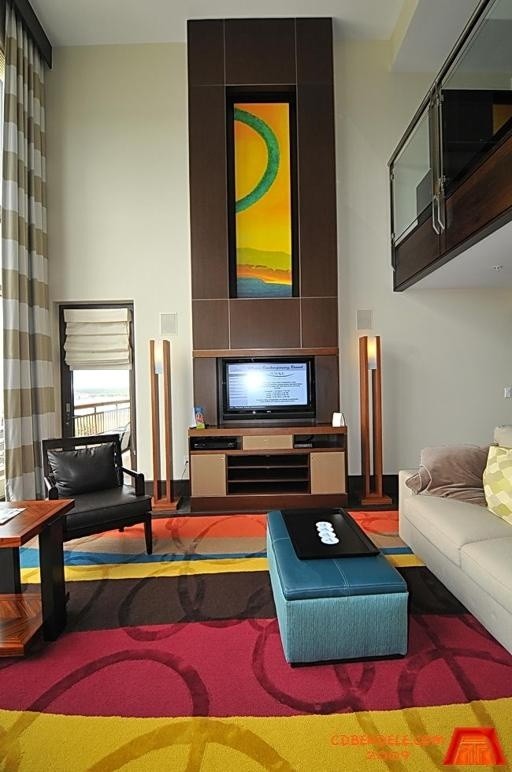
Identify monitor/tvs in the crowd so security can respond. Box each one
[222,359,317,420]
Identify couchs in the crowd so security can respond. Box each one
[396,423,512,659]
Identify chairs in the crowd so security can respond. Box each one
[93,420,130,457]
[35,433,156,557]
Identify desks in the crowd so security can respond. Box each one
[264,510,412,669]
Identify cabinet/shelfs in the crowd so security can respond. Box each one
[185,423,349,511]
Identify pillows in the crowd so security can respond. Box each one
[43,443,124,497]
[405,439,512,525]
